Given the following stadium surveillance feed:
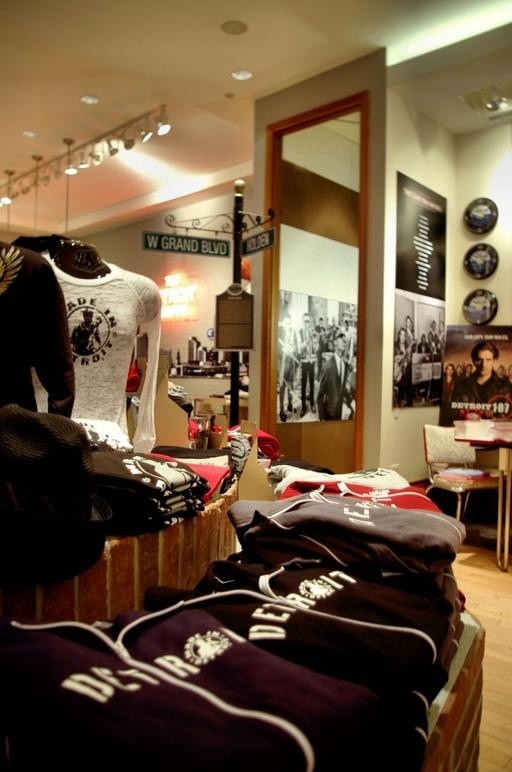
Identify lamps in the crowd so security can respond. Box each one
[0,103,171,209]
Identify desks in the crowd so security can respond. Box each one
[456,418,512,571]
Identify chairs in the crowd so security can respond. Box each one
[422,423,503,568]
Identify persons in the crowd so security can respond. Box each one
[30,239,162,456]
[0,240,77,421]
[277,304,511,421]
